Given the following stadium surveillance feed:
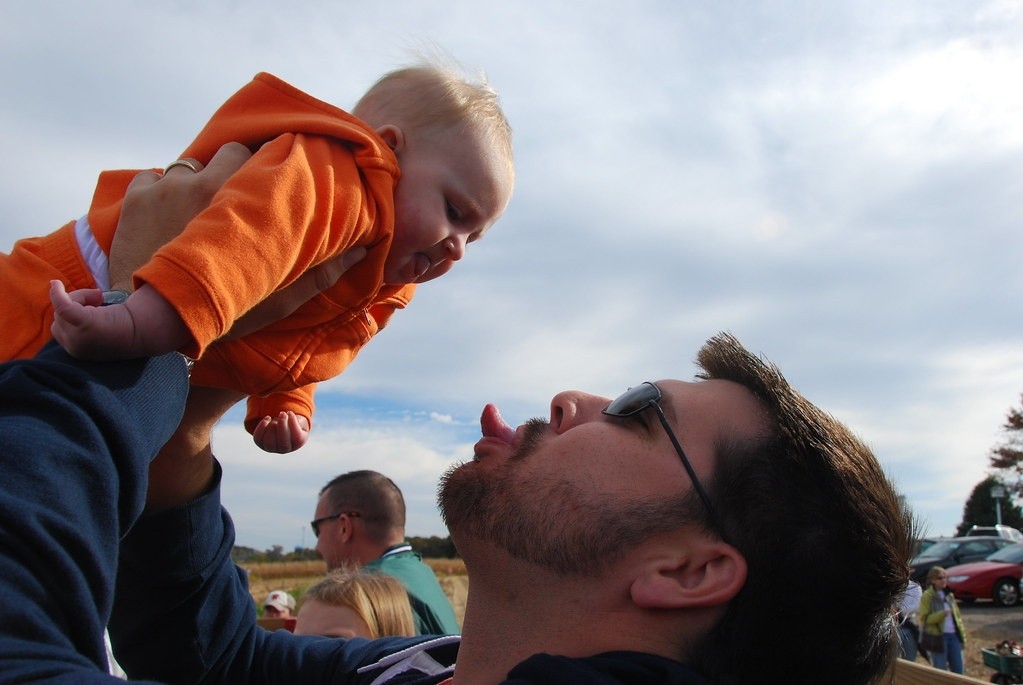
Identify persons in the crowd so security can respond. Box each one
[0,65,515,454]
[313,470,462,637]
[0,142,911,685]
[294,572,416,640]
[261,590,297,618]
[896,566,967,675]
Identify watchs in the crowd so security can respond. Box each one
[99,289,129,306]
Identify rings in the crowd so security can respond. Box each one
[163,161,198,175]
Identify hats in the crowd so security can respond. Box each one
[264,591,296,611]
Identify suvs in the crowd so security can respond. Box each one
[967,524,1023,543]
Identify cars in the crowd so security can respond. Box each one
[909,536,1014,584]
[943,543,1023,608]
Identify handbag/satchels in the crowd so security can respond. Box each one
[921,613,944,652]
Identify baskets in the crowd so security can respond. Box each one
[981,647,1023,674]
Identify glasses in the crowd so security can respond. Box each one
[601,382,730,544]
[936,576,946,580]
[311,512,360,537]
